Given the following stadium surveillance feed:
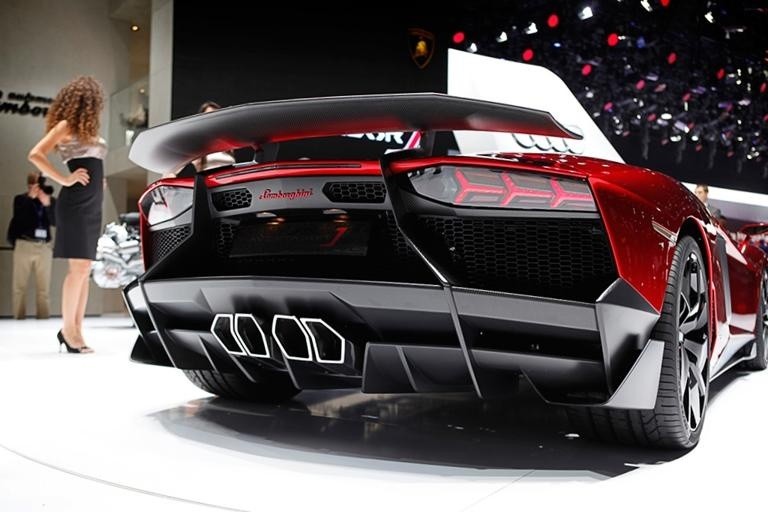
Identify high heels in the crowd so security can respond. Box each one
[57,328,95,353]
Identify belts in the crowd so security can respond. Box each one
[20,235,51,244]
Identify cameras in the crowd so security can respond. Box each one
[38,176,53,194]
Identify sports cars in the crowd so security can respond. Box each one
[119,92,768,455]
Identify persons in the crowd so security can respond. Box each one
[693,183,723,222]
[6,171,60,320]
[24,76,110,354]
[162,102,239,181]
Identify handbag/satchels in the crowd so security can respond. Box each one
[7,216,17,246]
